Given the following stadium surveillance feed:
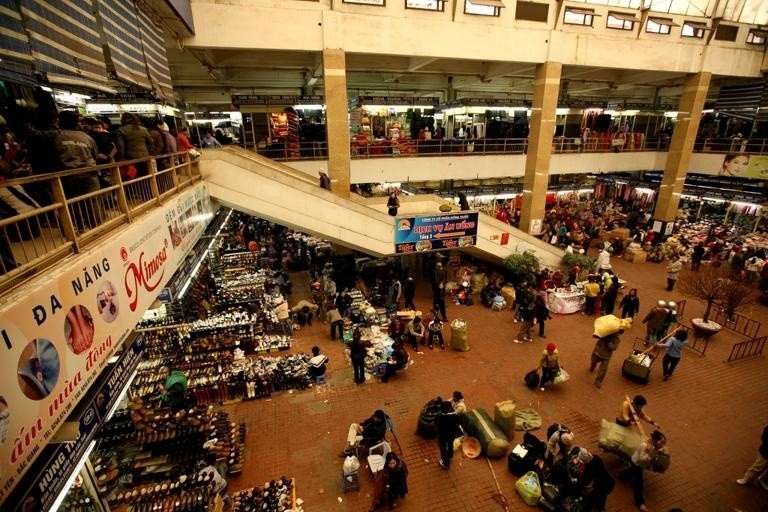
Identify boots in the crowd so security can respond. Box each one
[192,379,272,408]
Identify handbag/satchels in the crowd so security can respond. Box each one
[645,450,671,473]
[545,370,555,380]
[523,367,541,391]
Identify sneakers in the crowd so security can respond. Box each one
[438,456,452,470]
[539,387,545,391]
[511,316,549,344]
[736,474,767,490]
[413,342,446,352]
[65,395,295,512]
[131,253,314,400]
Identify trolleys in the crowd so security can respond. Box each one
[621,336,659,384]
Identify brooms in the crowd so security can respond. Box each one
[629,325,683,365]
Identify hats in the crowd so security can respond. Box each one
[453,390,464,399]
[561,433,574,445]
[667,301,676,309]
[546,342,556,352]
[657,299,667,307]
[578,448,593,464]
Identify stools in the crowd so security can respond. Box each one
[376,364,386,378]
[316,373,326,385]
[433,335,439,342]
[624,248,648,264]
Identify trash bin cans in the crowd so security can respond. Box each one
[611,139,620,153]
[730,138,748,152]
[702,138,713,152]
[573,139,581,150]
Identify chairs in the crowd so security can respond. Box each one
[395,351,410,373]
[366,441,392,480]
[541,277,558,294]
